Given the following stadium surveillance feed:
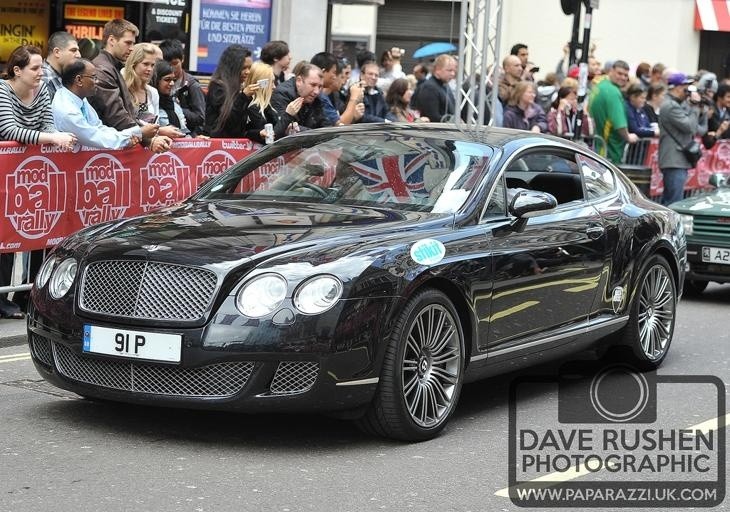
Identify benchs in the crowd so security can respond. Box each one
[506,173,584,205]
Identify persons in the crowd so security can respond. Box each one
[1,19,730,318]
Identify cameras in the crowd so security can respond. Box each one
[257,78,268,88]
[687,88,705,101]
[559,345,656,423]
[529,66,540,73]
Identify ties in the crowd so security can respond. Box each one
[81,101,88,123]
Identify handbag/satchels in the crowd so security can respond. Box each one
[682,140,703,163]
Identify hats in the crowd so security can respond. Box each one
[697,72,718,94]
[155,58,174,81]
[667,72,694,84]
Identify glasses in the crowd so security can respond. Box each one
[162,78,177,83]
[80,74,97,81]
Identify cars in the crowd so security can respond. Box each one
[28,123,688,444]
[668,171,730,299]
